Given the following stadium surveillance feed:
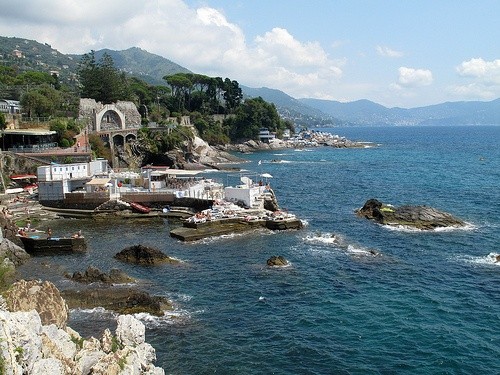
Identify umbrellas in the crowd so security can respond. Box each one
[261,174,273,181]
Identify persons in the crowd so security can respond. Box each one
[259,180,262,186]
[47,229,51,239]
[19,229,26,236]
